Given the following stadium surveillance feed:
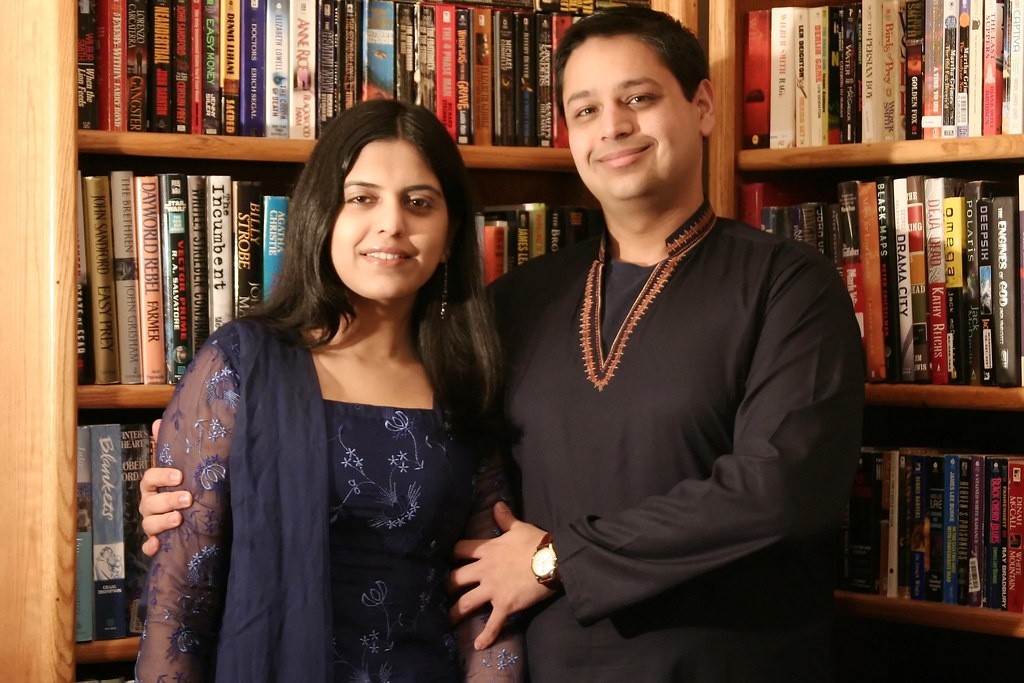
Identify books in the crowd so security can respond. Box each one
[77,0,1024,644]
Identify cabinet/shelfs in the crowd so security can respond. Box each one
[0,0,1024,683]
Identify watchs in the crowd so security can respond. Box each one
[531,532,561,591]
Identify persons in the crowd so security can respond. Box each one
[137,101,525,683]
[140,6,868,683]
